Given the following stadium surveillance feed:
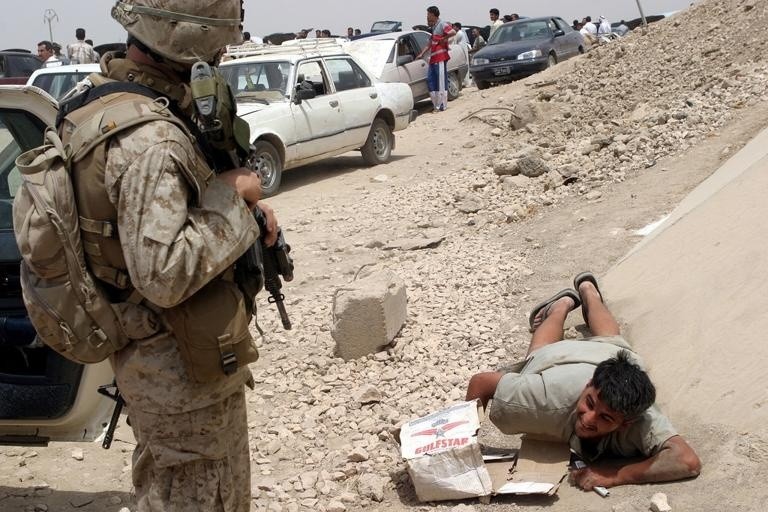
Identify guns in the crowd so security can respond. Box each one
[190,61,293,329]
[96,378,127,448]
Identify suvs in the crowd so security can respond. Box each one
[350,21,403,39]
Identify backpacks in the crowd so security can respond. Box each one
[10,82,195,365]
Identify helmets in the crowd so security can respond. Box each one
[102,0,254,75]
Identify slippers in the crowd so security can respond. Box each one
[526,287,583,334]
[574,267,603,328]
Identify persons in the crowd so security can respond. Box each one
[324,30,332,38]
[487,8,504,42]
[52,43,70,65]
[465,271,702,492]
[85,40,99,63]
[582,14,597,36]
[67,28,93,65]
[572,20,578,31]
[416,6,467,115]
[239,25,243,34]
[598,15,611,34]
[468,27,485,53]
[243,31,255,43]
[512,13,519,21]
[12,0,278,511]
[578,23,586,54]
[316,30,322,37]
[38,41,62,69]
[505,15,513,23]
[453,22,470,88]
[348,28,353,36]
[355,29,361,36]
[301,30,307,38]
[617,20,629,37]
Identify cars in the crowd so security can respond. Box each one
[215,49,418,199]
[0,48,42,85]
[24,62,103,103]
[0,84,120,449]
[469,16,585,90]
[348,31,466,101]
